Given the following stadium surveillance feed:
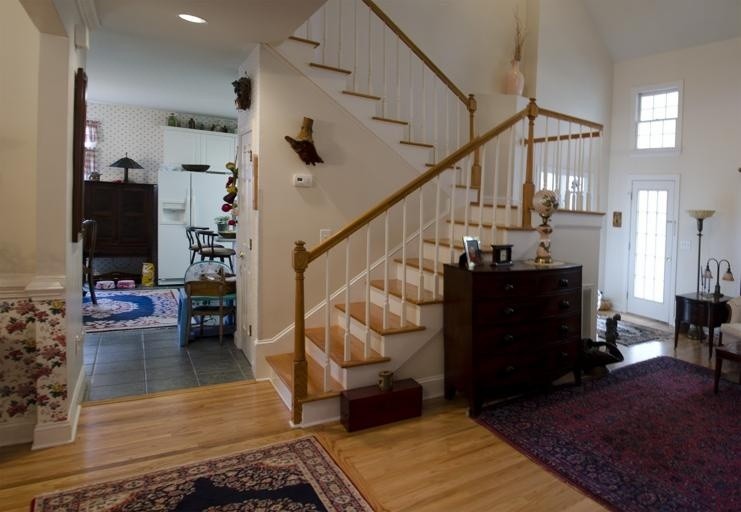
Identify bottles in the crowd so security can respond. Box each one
[167,112,178,127]
[188,117,196,129]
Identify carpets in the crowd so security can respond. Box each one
[81,287,180,333]
[597,314,673,346]
[30,436,375,511]
[470,356,741,511]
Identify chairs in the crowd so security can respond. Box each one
[185,280,237,346]
[81,219,98,306]
[186,226,236,277]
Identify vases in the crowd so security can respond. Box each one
[504,59,524,97]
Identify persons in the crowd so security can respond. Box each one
[468,243,480,262]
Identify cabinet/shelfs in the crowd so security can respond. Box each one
[441,259,582,419]
[160,124,236,174]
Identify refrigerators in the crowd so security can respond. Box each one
[158,171,238,285]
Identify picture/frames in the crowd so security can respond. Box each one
[462,235,484,268]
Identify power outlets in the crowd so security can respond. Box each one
[320,228,332,243]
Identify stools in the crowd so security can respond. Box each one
[340,377,422,432]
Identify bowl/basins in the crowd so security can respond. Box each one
[218,231,237,239]
[182,164,210,171]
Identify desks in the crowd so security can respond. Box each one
[713,322,741,393]
[674,291,734,359]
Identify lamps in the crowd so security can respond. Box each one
[685,211,716,339]
[108,152,144,182]
[701,258,734,297]
